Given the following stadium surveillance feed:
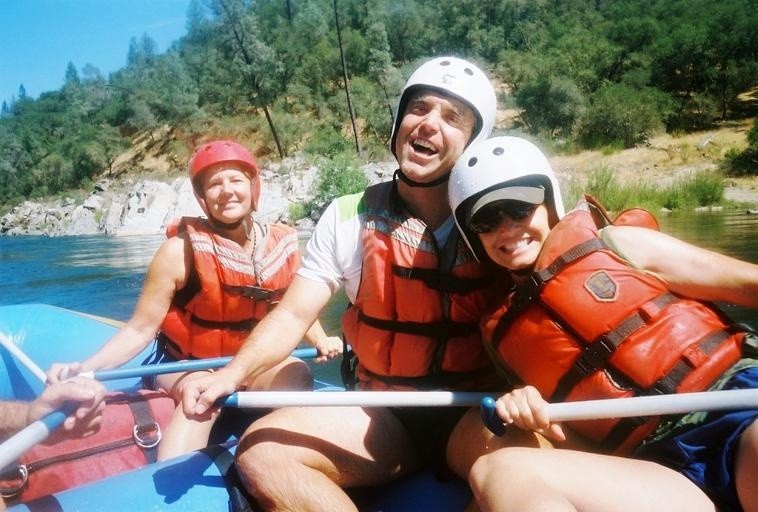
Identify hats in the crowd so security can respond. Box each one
[464,177,545,226]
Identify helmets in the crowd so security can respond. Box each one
[187,141,259,228]
[447,137,565,262]
[389,57,498,163]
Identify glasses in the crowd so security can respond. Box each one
[468,198,535,236]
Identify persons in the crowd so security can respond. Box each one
[448,136,758,512]
[181,56,517,512]
[45,136,348,463]
[0,377,107,512]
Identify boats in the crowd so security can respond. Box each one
[0,303,473,512]
